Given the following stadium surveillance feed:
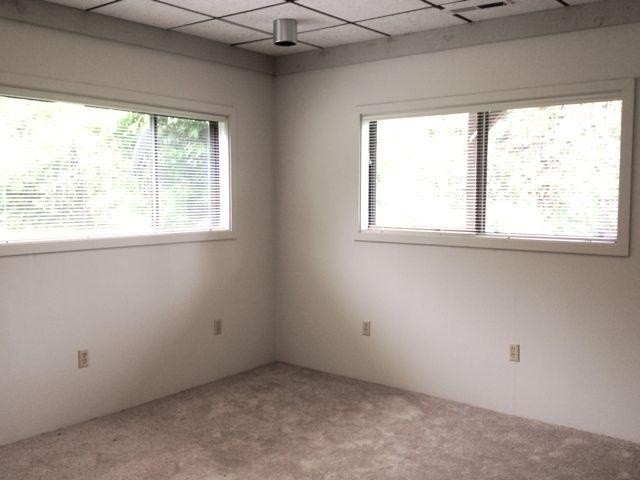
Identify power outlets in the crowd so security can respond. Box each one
[361,320,371,337]
[213,320,223,334]
[509,343,520,363]
[77,349,89,368]
